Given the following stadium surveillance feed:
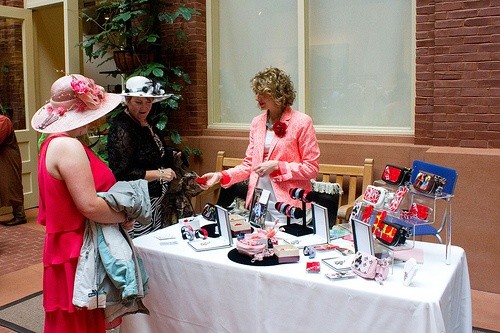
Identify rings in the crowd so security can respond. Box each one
[262,173,265,177]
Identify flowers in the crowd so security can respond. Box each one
[46,73,105,116]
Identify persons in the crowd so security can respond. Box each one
[31,74,135,332]
[107,76,189,240]
[0,105,27,226]
[196,67,320,224]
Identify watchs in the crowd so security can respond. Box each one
[120,209,130,225]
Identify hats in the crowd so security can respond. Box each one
[120,76,174,103]
[31,73,121,133]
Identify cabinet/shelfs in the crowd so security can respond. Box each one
[349,163,455,275]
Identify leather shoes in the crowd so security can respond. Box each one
[6,217,27,226]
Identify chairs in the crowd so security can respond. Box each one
[357,160,459,244]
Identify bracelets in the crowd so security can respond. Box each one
[181,225,208,241]
[157,169,164,182]
[277,188,313,220]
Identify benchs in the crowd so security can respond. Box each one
[189,151,374,225]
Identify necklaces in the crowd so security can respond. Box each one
[404,263,414,281]
[266,115,281,130]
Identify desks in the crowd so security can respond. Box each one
[120,214,472,333]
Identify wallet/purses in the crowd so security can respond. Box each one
[413,170,447,197]
[388,186,410,212]
[348,202,363,227]
[381,164,412,186]
[363,185,389,209]
[374,220,410,247]
[404,202,434,222]
[361,205,374,224]
[351,252,391,284]
